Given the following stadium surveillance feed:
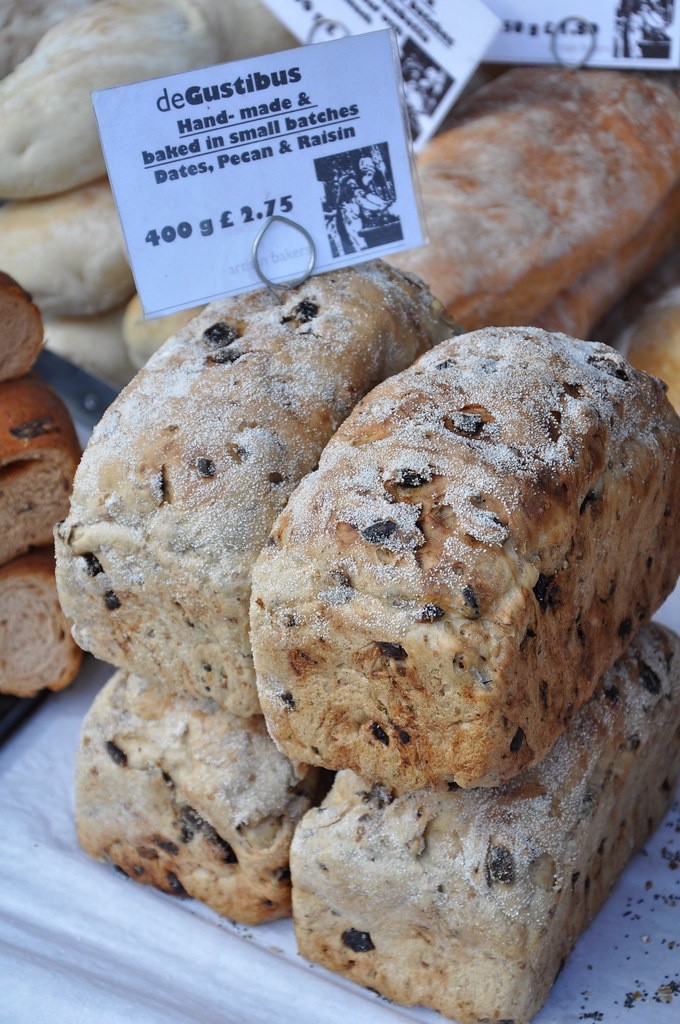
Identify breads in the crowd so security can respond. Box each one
[0,0,680,1024]
